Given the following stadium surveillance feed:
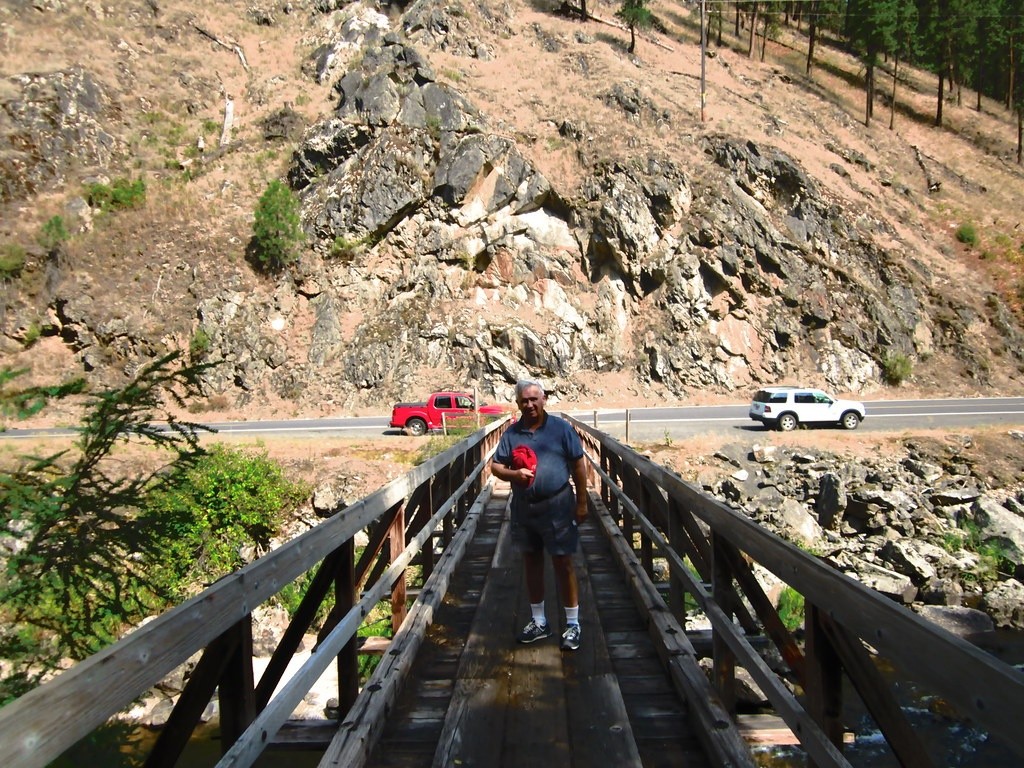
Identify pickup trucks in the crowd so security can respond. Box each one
[386,392,518,436]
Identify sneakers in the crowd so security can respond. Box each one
[561,624,582,651]
[516,618,552,644]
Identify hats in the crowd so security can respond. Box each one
[511,446,538,491]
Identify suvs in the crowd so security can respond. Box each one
[746,384,866,430]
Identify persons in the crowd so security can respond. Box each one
[491,380,590,650]
